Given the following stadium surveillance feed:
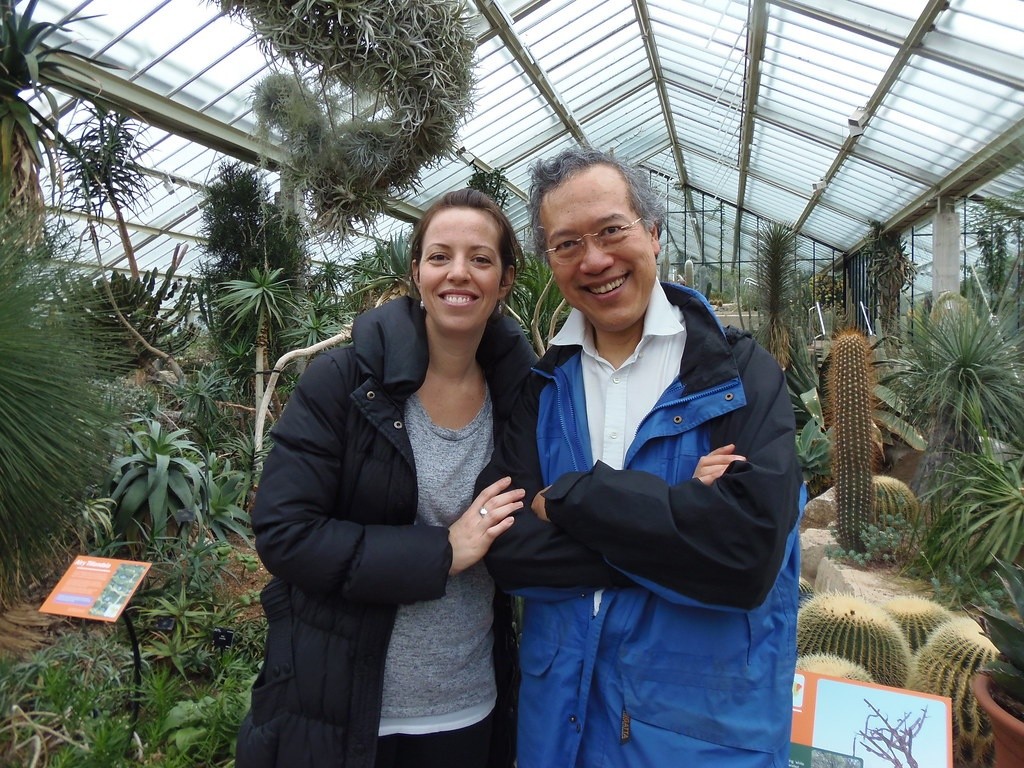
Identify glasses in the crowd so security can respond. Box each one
[544,217,644,266]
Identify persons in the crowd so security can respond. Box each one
[484,151,806,768]
[237,189,540,767]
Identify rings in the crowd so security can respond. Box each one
[479,508,486,517]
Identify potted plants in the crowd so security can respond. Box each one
[954,548,1024,768]
